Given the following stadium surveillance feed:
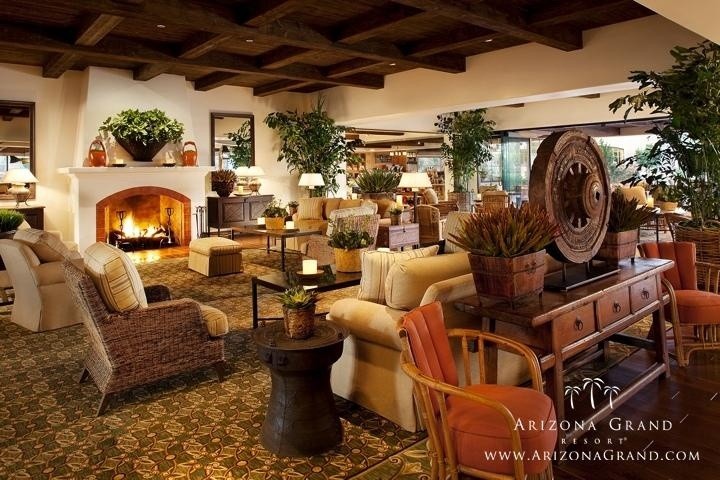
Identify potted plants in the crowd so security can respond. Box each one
[210,169,237,198]
[607,40,719,290]
[96,108,186,162]
[432,108,498,214]
[355,167,404,201]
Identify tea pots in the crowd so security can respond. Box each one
[181,141,198,165]
[88,141,107,167]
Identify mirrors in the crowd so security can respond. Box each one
[210,111,255,168]
[0,99,39,202]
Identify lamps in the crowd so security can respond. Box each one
[1,170,15,196]
[0,167,39,209]
[298,174,326,200]
[246,166,266,194]
[235,166,249,193]
[397,171,435,225]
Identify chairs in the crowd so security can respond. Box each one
[0,183,720,480]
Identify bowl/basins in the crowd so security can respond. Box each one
[296,269,325,279]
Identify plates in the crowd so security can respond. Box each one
[112,163,127,167]
[254,224,266,227]
[163,163,175,167]
[282,228,298,232]
[234,193,251,196]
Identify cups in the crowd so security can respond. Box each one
[397,195,402,204]
[257,218,266,224]
[352,193,357,199]
[116,159,123,164]
[237,186,243,193]
[286,221,295,229]
[303,260,318,275]
